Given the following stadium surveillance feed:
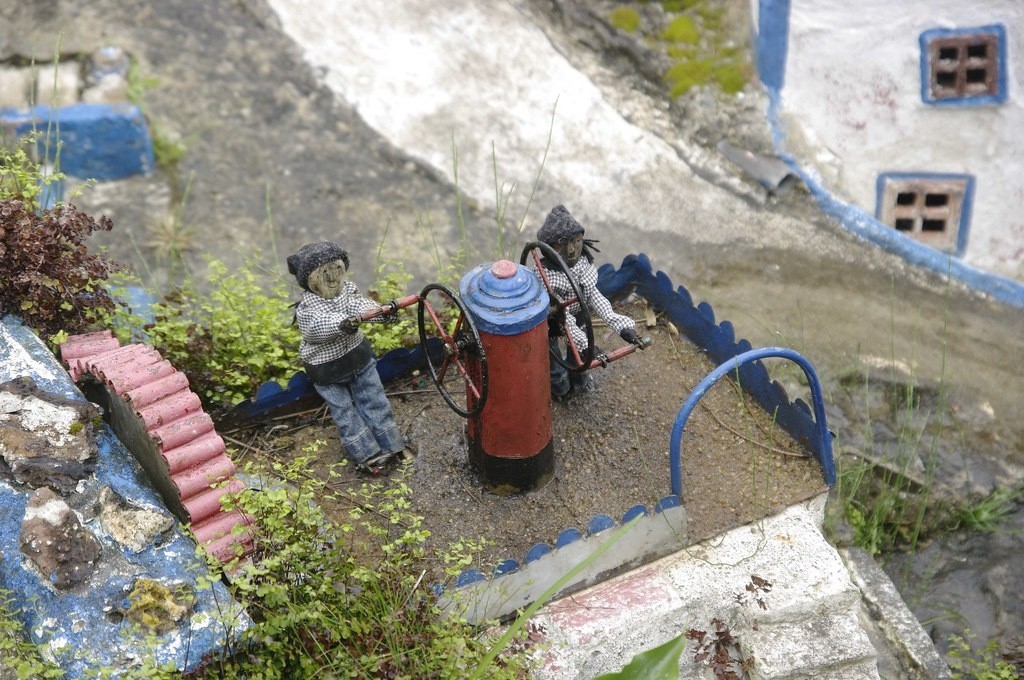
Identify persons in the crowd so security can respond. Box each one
[286,242,415,475]
[534,204,638,409]
